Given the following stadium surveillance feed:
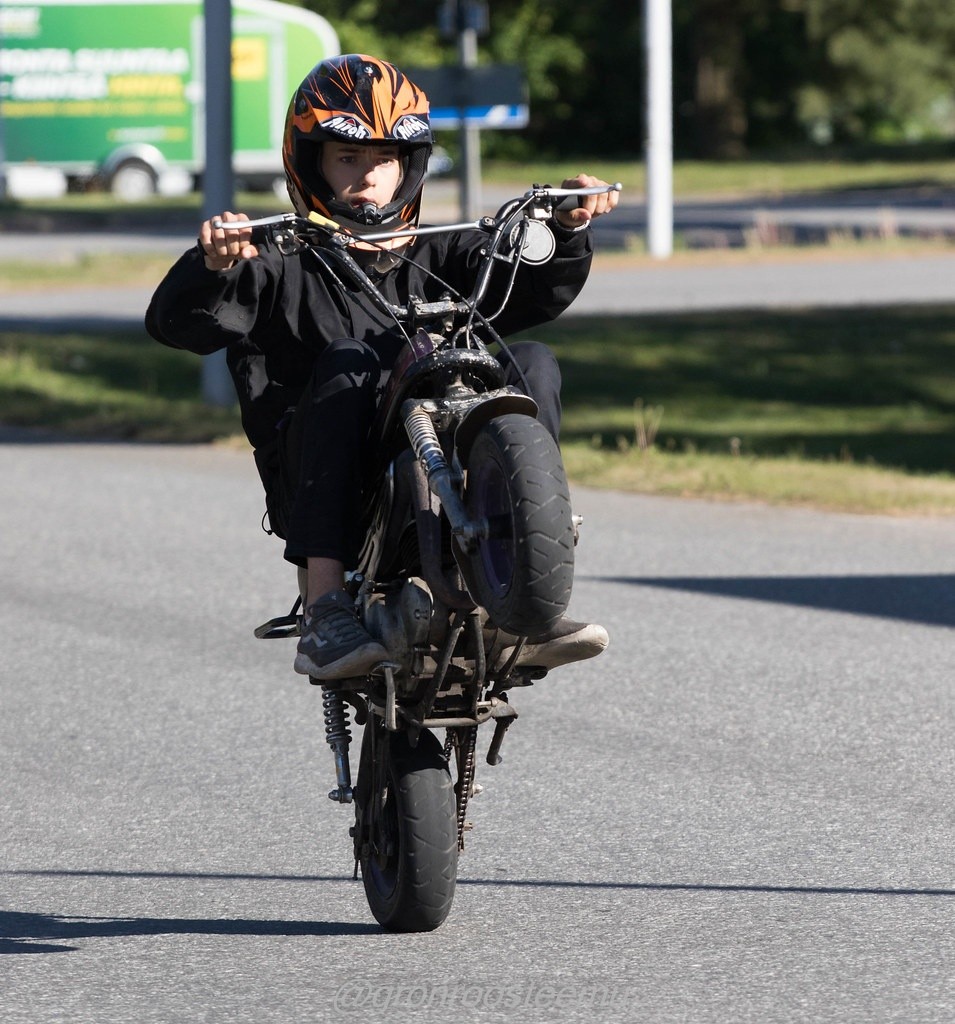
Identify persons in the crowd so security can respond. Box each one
[142,53,619,679]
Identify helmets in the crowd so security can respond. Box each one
[282,54,431,252]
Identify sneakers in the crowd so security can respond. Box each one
[464,613,609,671]
[294,589,388,677]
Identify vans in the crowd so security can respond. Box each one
[0,0,345,203]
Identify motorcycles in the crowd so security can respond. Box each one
[210,181,628,931]
